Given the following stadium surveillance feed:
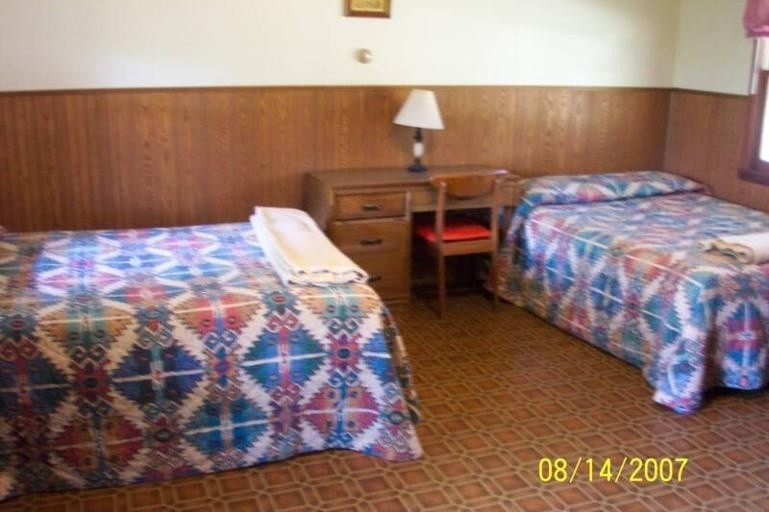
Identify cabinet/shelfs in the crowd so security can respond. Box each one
[303,165,520,313]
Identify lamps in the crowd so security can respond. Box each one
[393,88,445,171]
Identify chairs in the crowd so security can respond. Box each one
[415,170,514,318]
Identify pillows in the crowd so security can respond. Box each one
[522,171,708,206]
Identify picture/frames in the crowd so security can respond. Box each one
[346,0,391,18]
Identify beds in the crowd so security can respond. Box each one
[478,171,768,414]
[0,220,429,501]
